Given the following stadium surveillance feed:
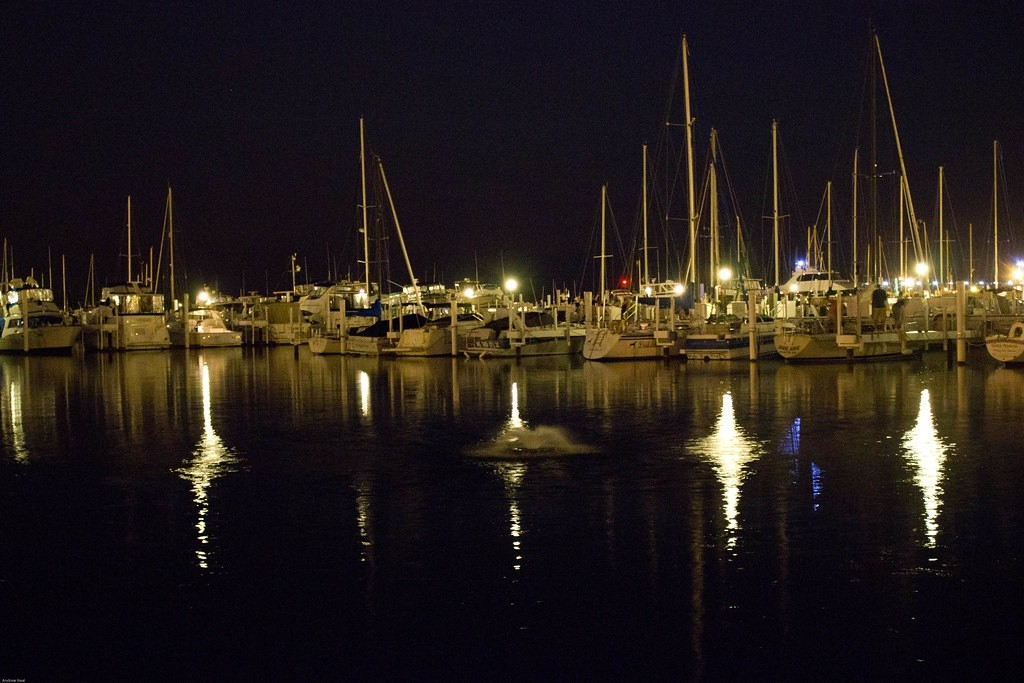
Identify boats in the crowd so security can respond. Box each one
[0,19,1023,361]
